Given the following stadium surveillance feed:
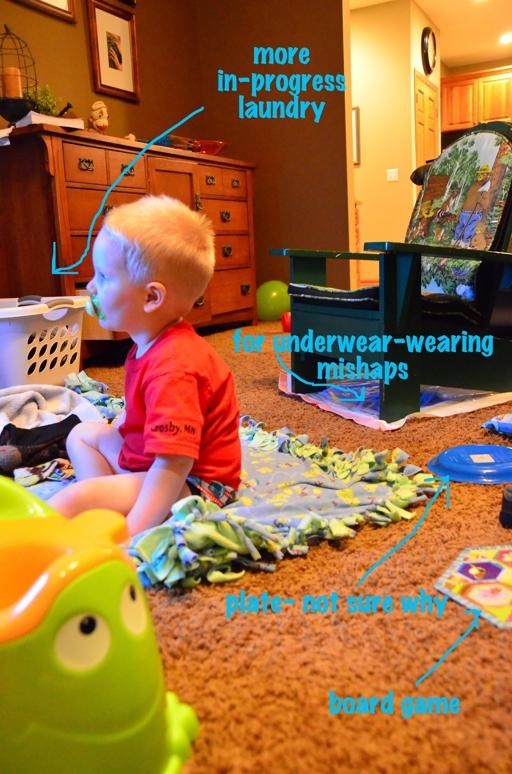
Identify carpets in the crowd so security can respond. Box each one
[275,366,511,433]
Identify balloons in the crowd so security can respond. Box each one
[257,279,291,321]
[89,101,110,133]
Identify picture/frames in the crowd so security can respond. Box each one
[8,0,78,25]
[86,0,142,103]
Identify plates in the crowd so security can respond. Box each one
[426,443,512,482]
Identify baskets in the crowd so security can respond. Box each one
[0,295,93,389]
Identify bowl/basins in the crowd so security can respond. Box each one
[200,139,226,155]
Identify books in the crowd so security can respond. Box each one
[0,469,200,773]
[15,111,85,129]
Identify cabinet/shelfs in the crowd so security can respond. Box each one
[0,122,259,372]
[440,65,512,133]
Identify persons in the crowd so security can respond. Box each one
[40,192,243,538]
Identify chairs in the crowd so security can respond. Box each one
[269,117,511,426]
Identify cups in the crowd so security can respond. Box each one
[281,311,290,331]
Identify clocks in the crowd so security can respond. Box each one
[422,25,438,75]
